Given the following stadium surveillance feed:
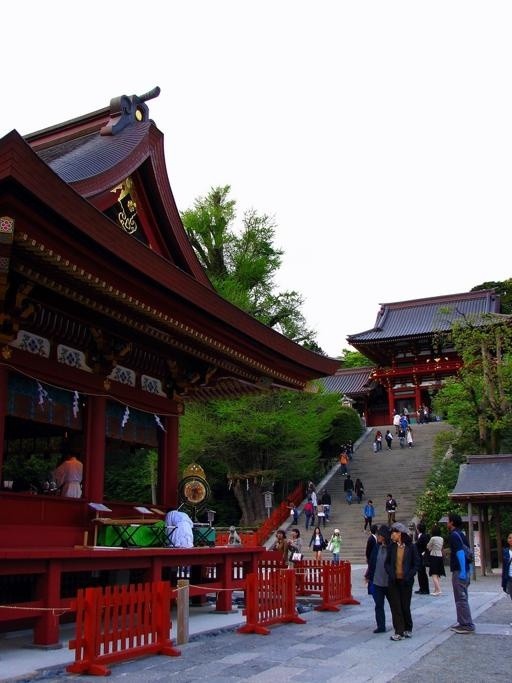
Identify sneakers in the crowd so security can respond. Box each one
[403,631,412,637]
[390,634,405,641]
[415,590,441,596]
[374,628,385,633]
[450,624,474,633]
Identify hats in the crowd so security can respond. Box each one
[391,522,408,533]
[334,529,340,533]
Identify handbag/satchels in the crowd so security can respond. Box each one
[464,544,473,564]
[326,543,334,551]
[322,539,328,550]
[292,552,301,561]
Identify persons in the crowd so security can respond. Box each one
[228,526,242,544]
[54,449,83,499]
[165,507,193,548]
[274,402,512,640]
